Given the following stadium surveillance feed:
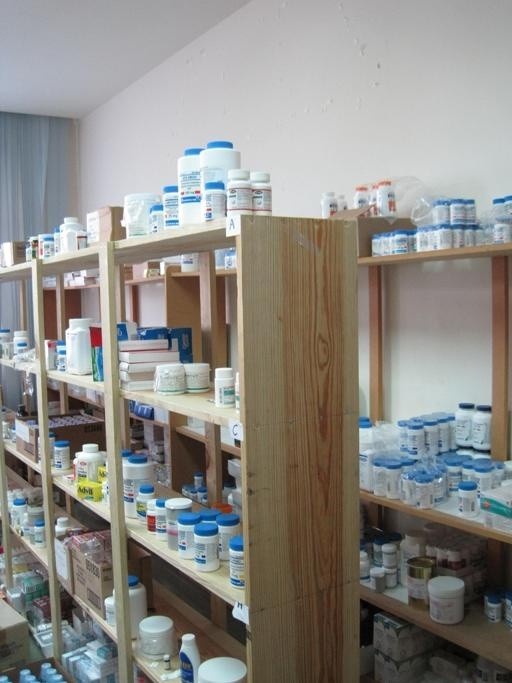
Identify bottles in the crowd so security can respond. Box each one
[156,361,211,395]
[320,179,511,257]
[213,366,241,411]
[0,325,31,357]
[0,660,69,683]
[2,485,74,549]
[56,340,66,371]
[357,400,512,517]
[65,317,96,375]
[23,138,272,273]
[48,431,246,590]
[356,521,512,630]
[105,573,202,682]
[18,403,29,416]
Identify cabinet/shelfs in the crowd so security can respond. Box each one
[357,242,511,683]
[1,213,362,682]
